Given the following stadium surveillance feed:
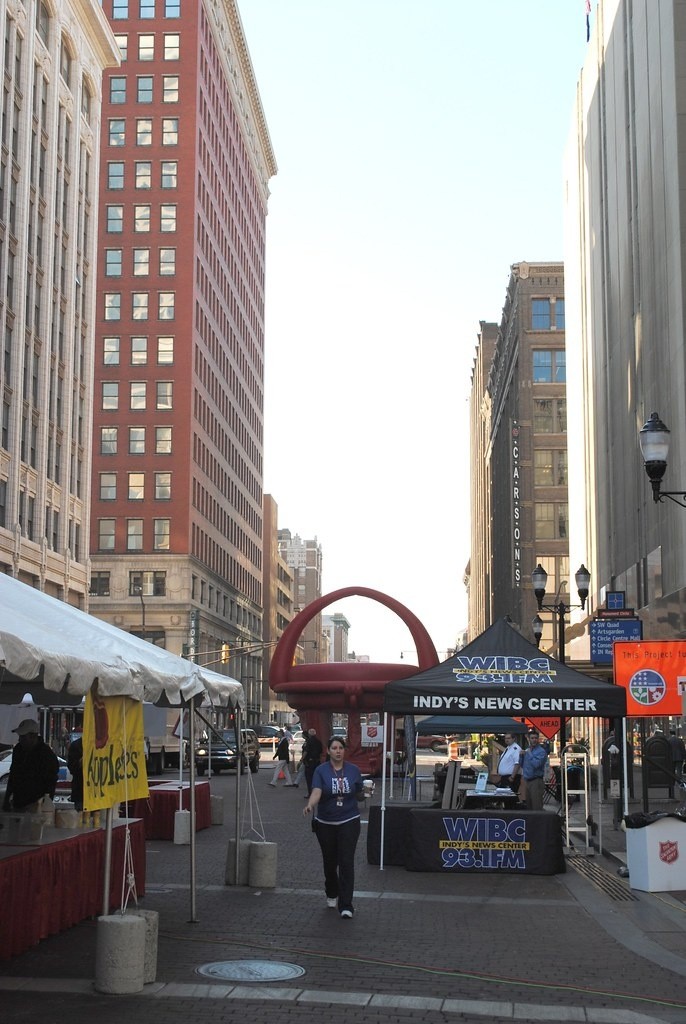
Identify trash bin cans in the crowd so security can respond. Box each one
[552,765,582,802]
[626,812,686,893]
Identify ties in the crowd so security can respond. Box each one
[497,748,508,773]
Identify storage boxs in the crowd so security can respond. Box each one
[0,812,48,841]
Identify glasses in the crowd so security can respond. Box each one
[328,735,343,740]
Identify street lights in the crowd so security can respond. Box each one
[531,563,592,757]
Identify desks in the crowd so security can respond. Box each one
[0,817,145,963]
[120,780,211,840]
[457,782,498,809]
[466,789,517,810]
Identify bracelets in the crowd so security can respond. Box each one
[511,776,515,779]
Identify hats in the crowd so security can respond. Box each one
[12,719,39,735]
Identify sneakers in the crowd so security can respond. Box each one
[326,897,337,909]
[341,910,353,918]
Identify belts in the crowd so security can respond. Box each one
[527,776,543,782]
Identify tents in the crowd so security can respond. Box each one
[408,716,528,798]
[380,619,628,870]
[0,566,248,923]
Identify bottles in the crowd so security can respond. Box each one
[37,793,55,827]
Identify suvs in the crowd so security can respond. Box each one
[195,728,261,776]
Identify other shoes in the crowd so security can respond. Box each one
[283,784,293,787]
[304,793,311,798]
[293,784,299,787]
[268,783,276,787]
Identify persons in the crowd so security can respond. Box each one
[267,731,293,788]
[519,730,547,810]
[283,726,293,742]
[5,718,60,807]
[303,736,373,918]
[67,737,101,816]
[496,731,523,801]
[293,726,322,799]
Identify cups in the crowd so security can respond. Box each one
[363,780,373,798]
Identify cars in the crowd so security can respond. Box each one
[396,727,447,753]
[330,713,381,743]
[288,731,307,753]
[254,725,282,749]
[263,721,303,738]
[0,748,74,787]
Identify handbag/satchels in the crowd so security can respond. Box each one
[683,761,686,773]
